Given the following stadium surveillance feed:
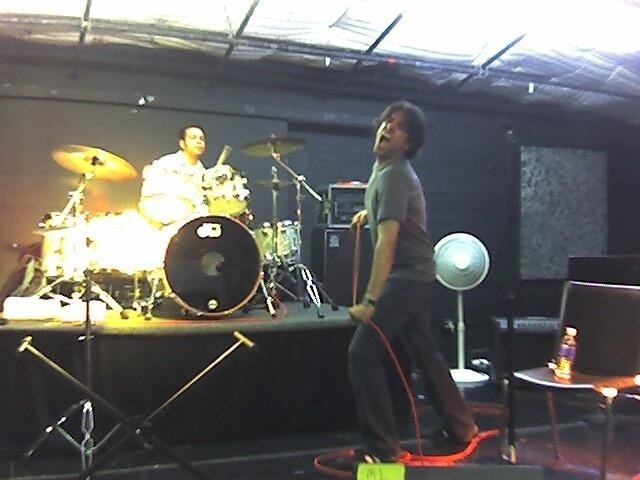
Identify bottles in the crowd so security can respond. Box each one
[554,326,577,381]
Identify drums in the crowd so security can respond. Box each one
[252,219,298,263]
[163,214,262,316]
[199,165,251,215]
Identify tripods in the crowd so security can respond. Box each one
[21,156,338,480]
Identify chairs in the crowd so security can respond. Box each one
[505,277,640,479]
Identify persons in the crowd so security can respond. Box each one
[346,100,481,463]
[138,125,206,228]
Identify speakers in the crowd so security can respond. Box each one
[311,226,378,306]
[319,184,368,227]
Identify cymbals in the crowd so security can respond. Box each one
[240,137,305,157]
[50,144,137,182]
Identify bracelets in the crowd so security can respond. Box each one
[362,298,378,307]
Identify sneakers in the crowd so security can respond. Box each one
[312,447,399,480]
